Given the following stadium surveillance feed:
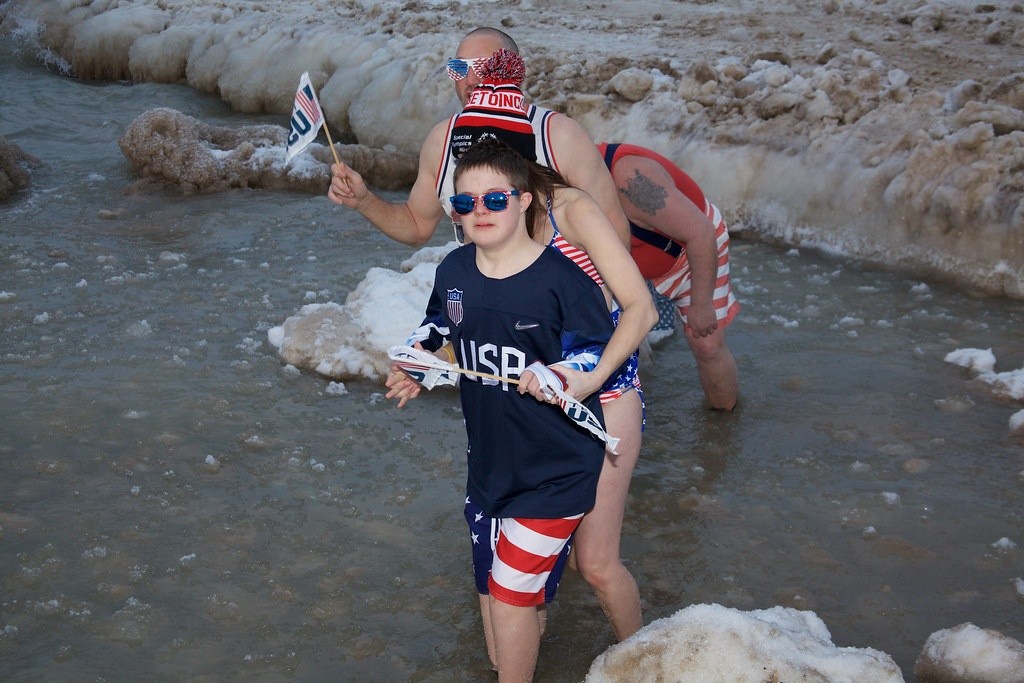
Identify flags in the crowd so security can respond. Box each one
[283,73,327,169]
[540,384,621,456]
[385,345,460,391]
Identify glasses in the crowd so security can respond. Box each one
[446,58,491,80]
[449,188,520,216]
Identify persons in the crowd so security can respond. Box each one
[324,28,633,258]
[449,51,657,645]
[598,143,741,425]
[384,141,617,683]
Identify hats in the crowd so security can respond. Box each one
[450,48,537,163]
